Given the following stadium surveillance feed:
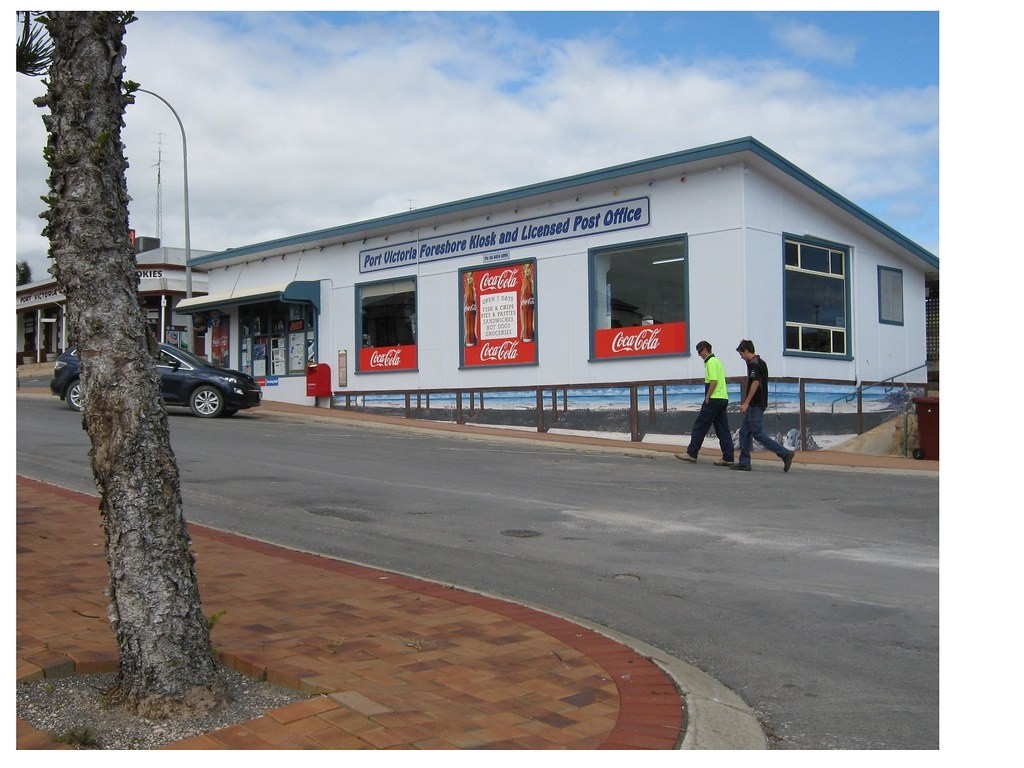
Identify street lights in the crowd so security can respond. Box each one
[122,81,195,354]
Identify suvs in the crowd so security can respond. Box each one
[48,338,263,418]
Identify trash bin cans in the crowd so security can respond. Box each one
[910,397,939,460]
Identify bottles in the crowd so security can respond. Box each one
[520,264,534,343]
[463,272,478,347]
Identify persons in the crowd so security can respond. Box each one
[676,341,734,467]
[726,339,794,473]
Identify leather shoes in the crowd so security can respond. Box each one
[783,450,795,472]
[713,460,735,467]
[730,464,751,471]
[676,453,698,463]
